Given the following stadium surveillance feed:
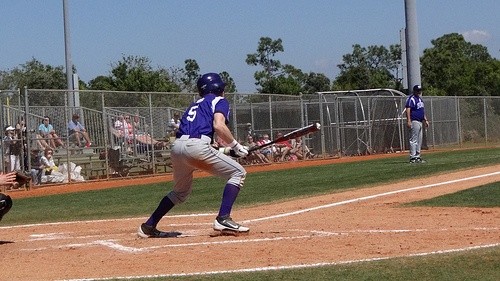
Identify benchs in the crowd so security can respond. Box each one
[56,146,173,179]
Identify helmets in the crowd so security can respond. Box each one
[197,73,224,97]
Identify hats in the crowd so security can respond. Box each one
[73,114,79,119]
[6,126,15,131]
[413,85,422,91]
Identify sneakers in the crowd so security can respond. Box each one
[138,223,168,238]
[213,214,249,233]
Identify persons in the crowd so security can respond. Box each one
[0,111,182,222]
[213,129,315,166]
[405,85,430,163]
[138,73,250,238]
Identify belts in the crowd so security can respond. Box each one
[176,134,201,138]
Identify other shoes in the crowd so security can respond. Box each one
[410,157,425,163]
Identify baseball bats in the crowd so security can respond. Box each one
[248,122,321,152]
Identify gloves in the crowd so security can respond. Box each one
[219,147,232,155]
[227,139,249,157]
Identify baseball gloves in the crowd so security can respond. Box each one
[423,121,429,129]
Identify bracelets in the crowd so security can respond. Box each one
[425,120,427,121]
